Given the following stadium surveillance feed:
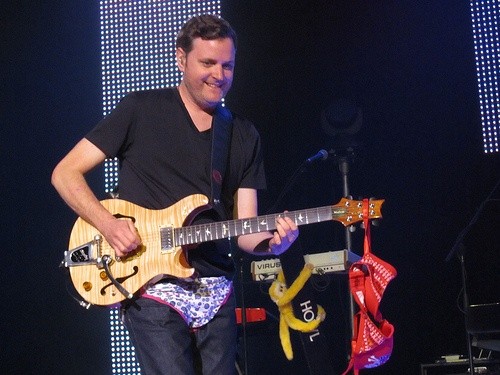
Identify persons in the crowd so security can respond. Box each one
[51,14,299,375]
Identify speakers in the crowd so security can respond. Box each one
[234,272,361,375]
[465,303,500,337]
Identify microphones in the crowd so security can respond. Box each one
[307,149,329,163]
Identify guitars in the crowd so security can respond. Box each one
[61,193,385,309]
